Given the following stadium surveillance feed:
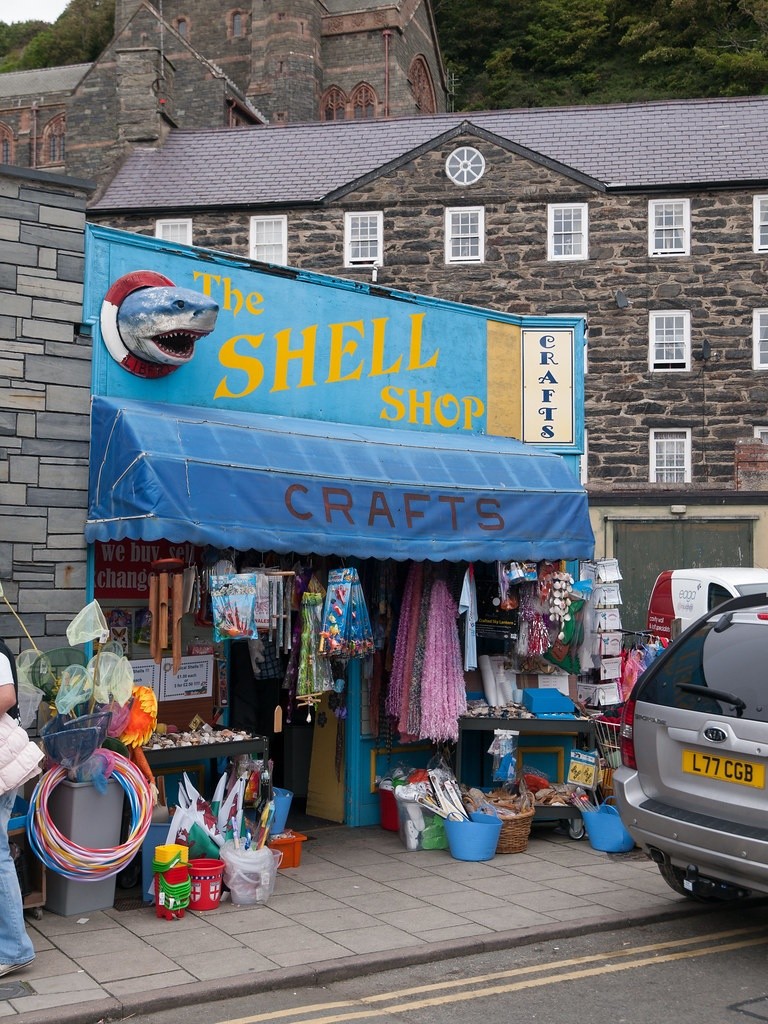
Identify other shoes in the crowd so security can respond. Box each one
[0,958,34,977]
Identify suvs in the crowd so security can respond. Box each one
[612,592,768,898]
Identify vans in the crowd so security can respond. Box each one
[646,567,768,645]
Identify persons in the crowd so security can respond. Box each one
[1,638,36,977]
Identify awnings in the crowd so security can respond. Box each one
[87,395,598,561]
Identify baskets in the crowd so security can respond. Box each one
[463,792,535,854]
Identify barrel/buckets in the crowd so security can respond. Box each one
[152,844,192,921]
[188,858,225,911]
[377,788,401,831]
